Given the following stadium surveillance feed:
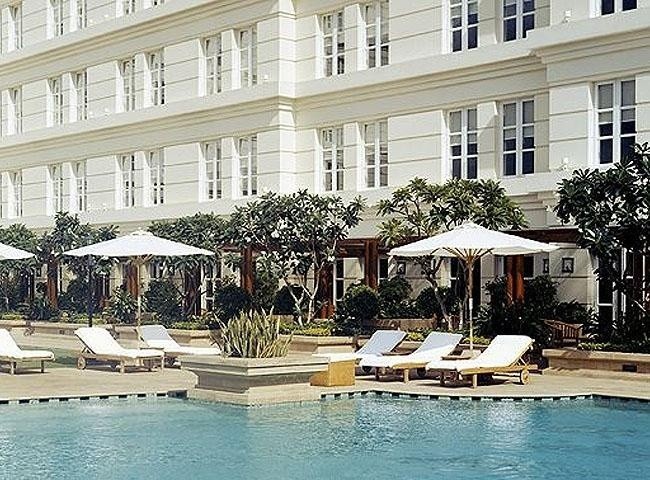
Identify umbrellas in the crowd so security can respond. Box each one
[62,229,216,348]
[385,221,561,353]
[0,240,36,264]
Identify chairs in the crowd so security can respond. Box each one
[545,316,583,347]
[74,324,220,376]
[0,328,56,374]
[314,325,541,390]
